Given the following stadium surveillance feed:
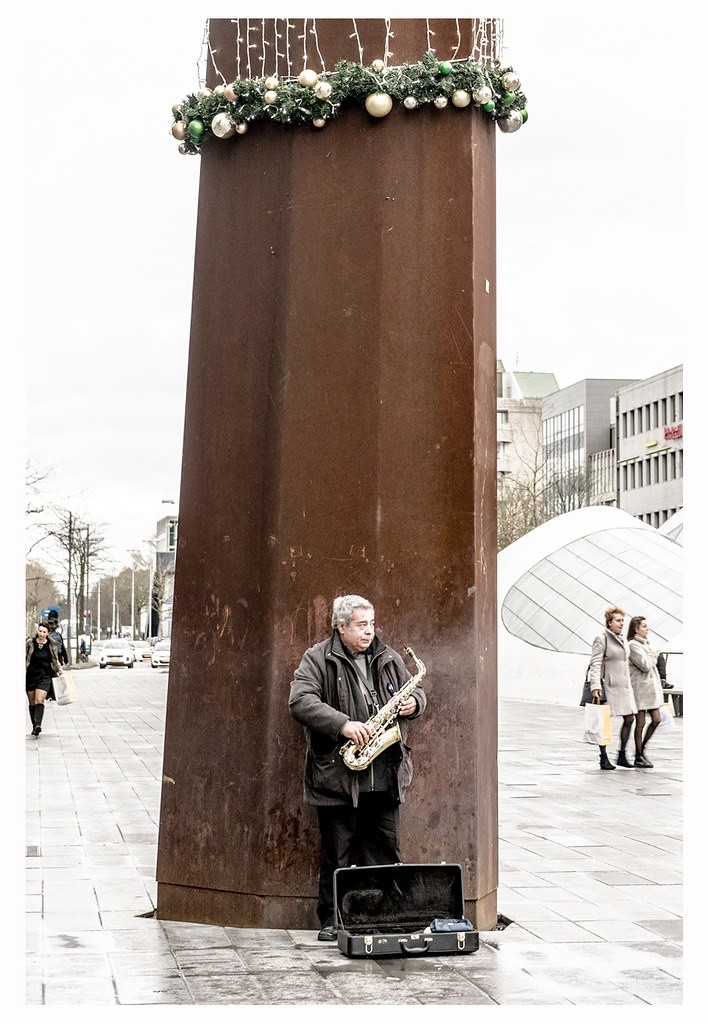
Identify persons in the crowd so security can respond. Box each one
[288,595,428,941]
[44,610,68,701]
[80,639,86,654]
[25,622,64,736]
[590,607,638,771]
[625,616,664,768]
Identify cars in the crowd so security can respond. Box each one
[97,639,136,668]
[91,635,165,662]
[150,640,170,669]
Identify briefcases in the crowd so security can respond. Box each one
[332,861,480,956]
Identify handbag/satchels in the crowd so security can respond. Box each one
[656,703,675,727]
[52,676,74,706]
[583,697,612,745]
[656,653,666,680]
[580,681,607,706]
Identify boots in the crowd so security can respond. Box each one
[617,751,635,768]
[33,704,45,736]
[29,705,35,734]
[599,754,616,769]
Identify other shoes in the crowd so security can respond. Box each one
[634,754,653,768]
[318,926,337,940]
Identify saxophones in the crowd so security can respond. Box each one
[338,643,427,771]
